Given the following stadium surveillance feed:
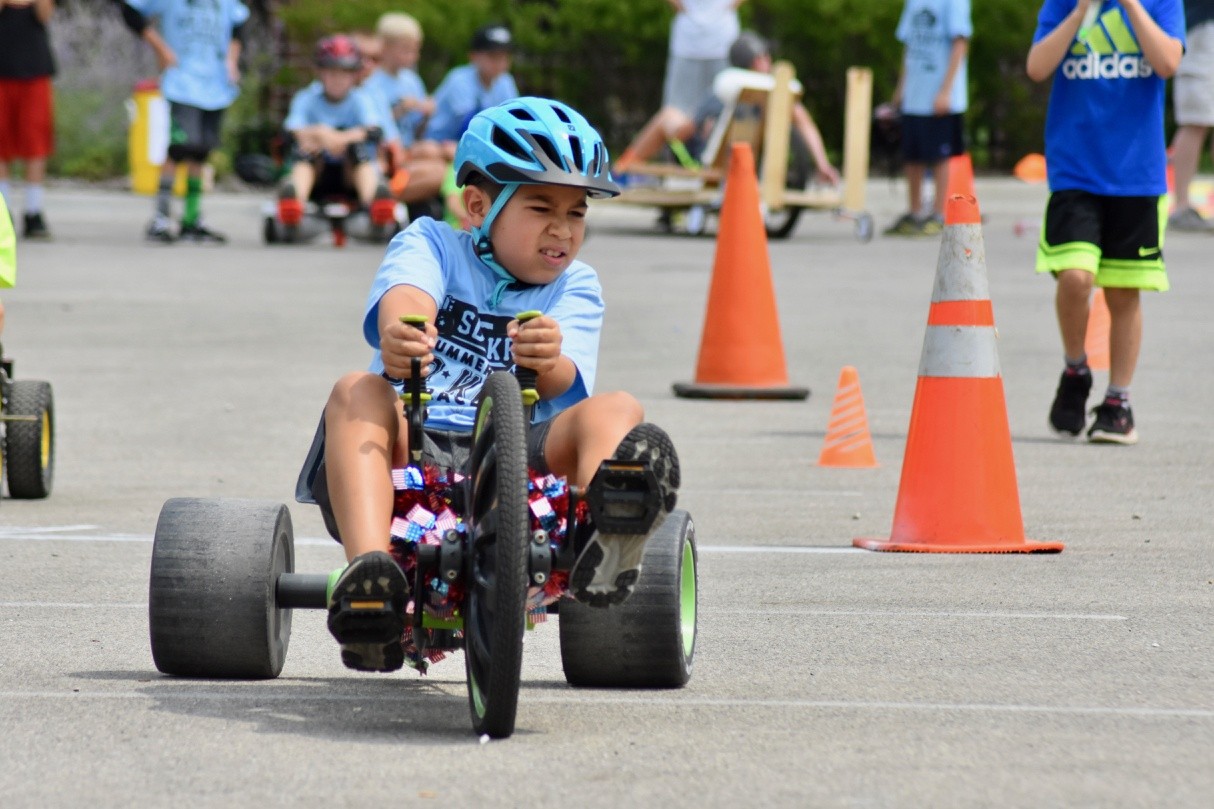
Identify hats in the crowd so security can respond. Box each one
[470,24,518,52]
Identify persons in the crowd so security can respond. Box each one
[279,9,520,232]
[611,0,841,227]
[294,98,681,674]
[116,0,266,246]
[0,192,17,336]
[1028,0,1214,442]
[875,0,975,236]
[0,0,60,237]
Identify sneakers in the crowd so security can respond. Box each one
[325,553,410,674]
[1049,359,1095,436]
[1089,394,1136,445]
[569,423,681,607]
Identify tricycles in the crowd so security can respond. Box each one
[148,309,701,741]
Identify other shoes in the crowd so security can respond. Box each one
[371,183,396,227]
[918,213,947,234]
[146,219,172,242]
[22,211,49,240]
[883,214,918,235]
[276,190,301,228]
[178,219,222,242]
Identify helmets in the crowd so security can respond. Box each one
[454,95,619,201]
[311,37,363,70]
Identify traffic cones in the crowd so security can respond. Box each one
[815,363,883,469]
[672,137,812,399]
[852,152,1066,555]
